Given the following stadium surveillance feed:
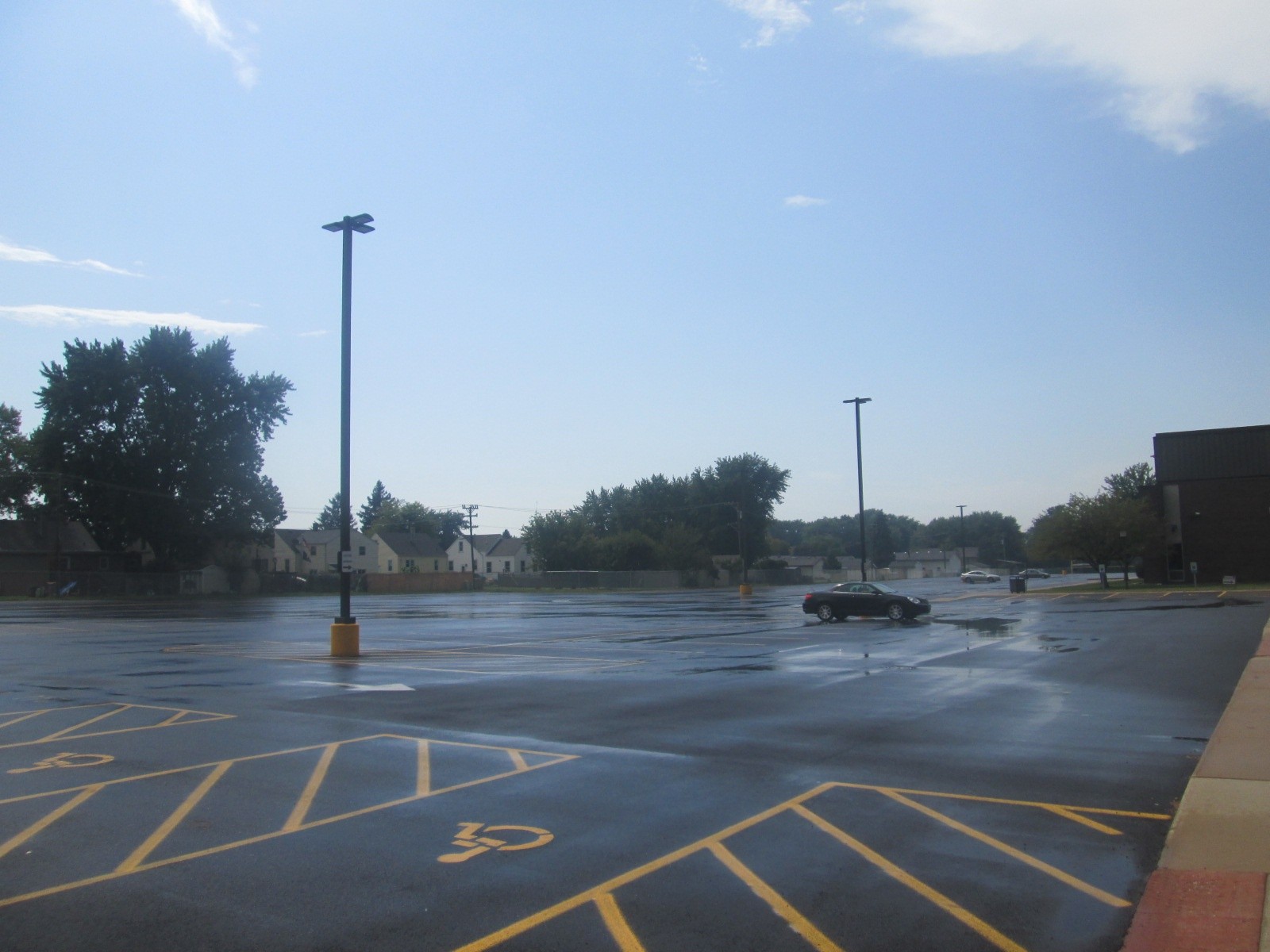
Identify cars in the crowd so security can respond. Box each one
[960,568,1001,584]
[802,580,930,627]
[1019,567,1050,579]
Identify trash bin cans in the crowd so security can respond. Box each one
[1009,575,1026,594]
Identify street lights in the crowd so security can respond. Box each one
[956,504,969,574]
[319,209,379,664]
[839,393,874,583]
